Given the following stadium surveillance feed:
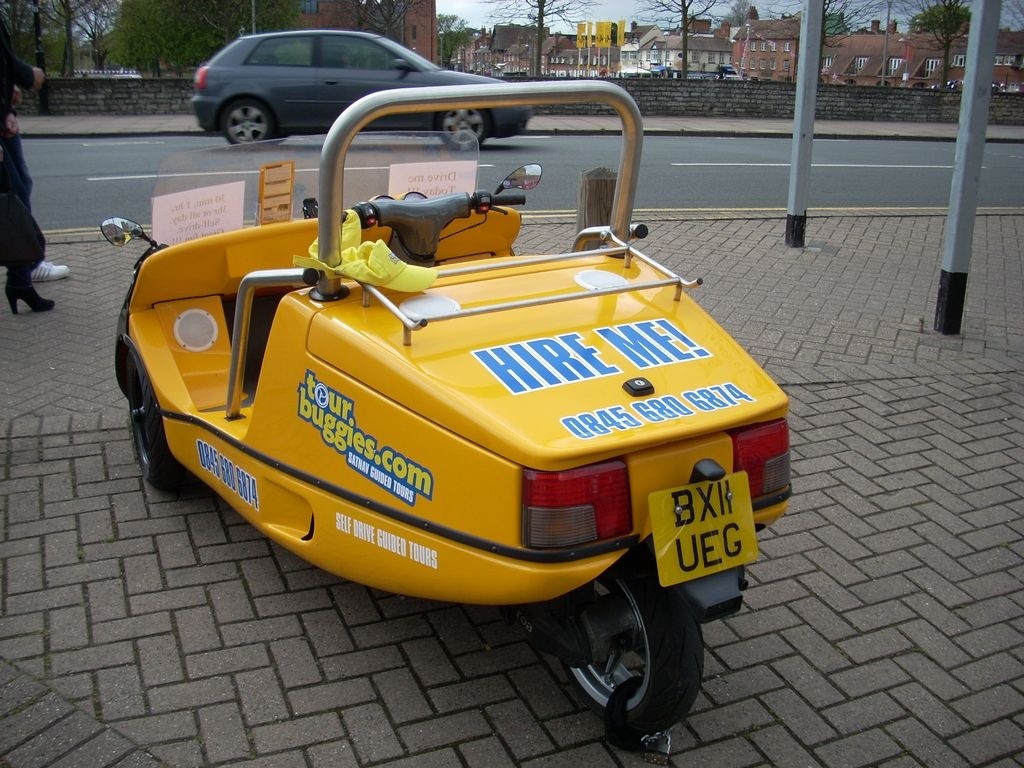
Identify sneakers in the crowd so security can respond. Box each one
[30,262,72,281]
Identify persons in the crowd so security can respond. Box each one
[0,0,71,315]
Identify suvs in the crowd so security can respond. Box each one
[191,29,532,150]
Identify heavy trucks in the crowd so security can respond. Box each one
[671,65,737,79]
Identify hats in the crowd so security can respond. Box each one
[293,239,438,292]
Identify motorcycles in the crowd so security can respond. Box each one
[101,75,793,730]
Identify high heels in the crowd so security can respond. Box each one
[5,283,55,314]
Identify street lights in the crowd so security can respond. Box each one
[473,33,480,70]
[664,32,670,76]
[438,31,444,68]
[897,38,909,87]
